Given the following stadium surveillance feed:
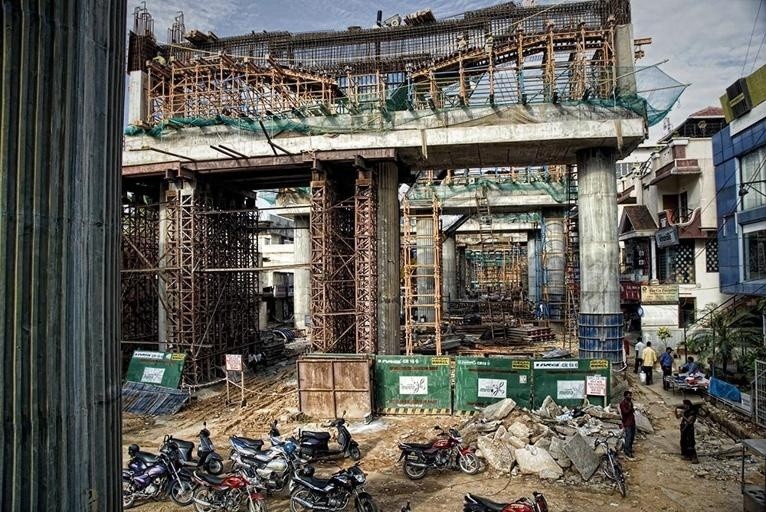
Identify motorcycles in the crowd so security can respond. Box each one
[397,424,480,479]
[463,491,548,512]
[289,458,378,512]
[123,410,360,512]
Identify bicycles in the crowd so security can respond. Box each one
[593,431,626,498]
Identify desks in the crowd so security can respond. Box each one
[666,375,703,399]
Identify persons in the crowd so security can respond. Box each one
[619,390,639,461]
[633,337,646,373]
[641,341,657,385]
[680,355,698,376]
[660,346,675,390]
[674,399,699,463]
